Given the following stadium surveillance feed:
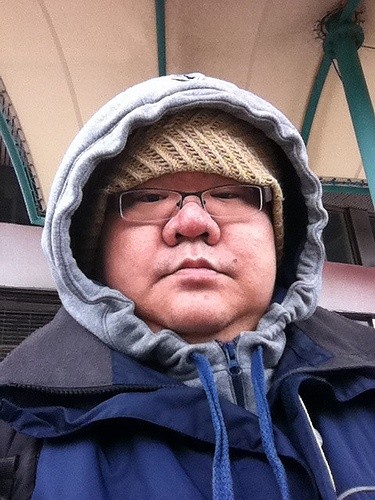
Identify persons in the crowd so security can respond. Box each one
[1,69,375,500]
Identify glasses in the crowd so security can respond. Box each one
[117,183,265,222]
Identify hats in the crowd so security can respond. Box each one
[83,109,287,269]
